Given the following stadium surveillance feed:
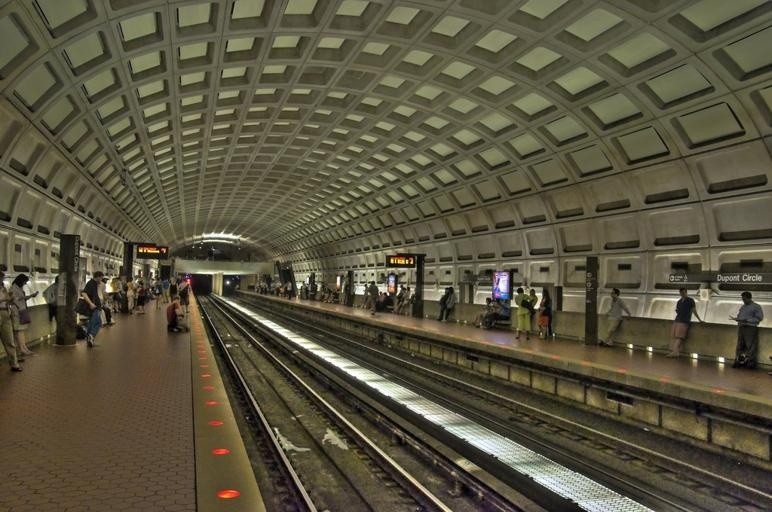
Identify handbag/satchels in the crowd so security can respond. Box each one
[19,309,31,324]
[732,346,753,369]
[172,311,179,327]
[521,299,530,308]
[73,298,92,316]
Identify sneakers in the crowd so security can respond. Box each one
[87,333,94,348]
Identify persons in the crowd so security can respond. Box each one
[0,271,24,372]
[596,288,631,347]
[472,287,554,341]
[9,274,38,356]
[253,278,416,317]
[664,287,705,358]
[436,287,456,322]
[43,270,190,349]
[731,291,763,371]
